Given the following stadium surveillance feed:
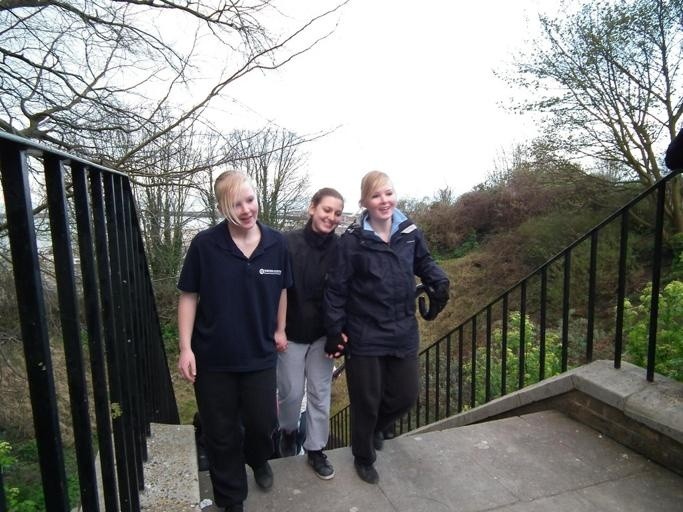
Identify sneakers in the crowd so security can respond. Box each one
[373,431,384,450]
[308,447,335,479]
[279,431,297,457]
[354,458,379,483]
[254,463,273,489]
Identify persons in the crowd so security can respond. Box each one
[175,169,295,511]
[323,169,451,486]
[273,187,350,481]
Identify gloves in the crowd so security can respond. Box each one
[433,285,450,313]
[324,334,347,359]
